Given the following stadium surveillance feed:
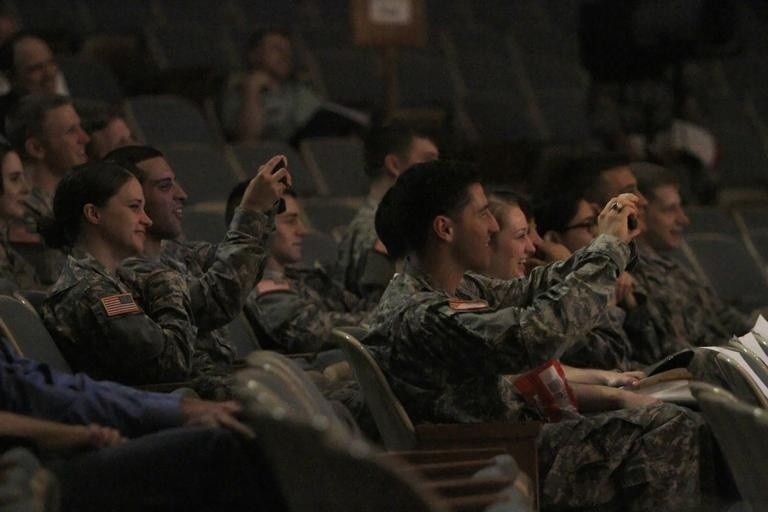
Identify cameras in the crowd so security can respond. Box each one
[270,159,288,193]
[626,212,636,229]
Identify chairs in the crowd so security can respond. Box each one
[0,0,768,512]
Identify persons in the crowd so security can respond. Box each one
[0,1,768,512]
